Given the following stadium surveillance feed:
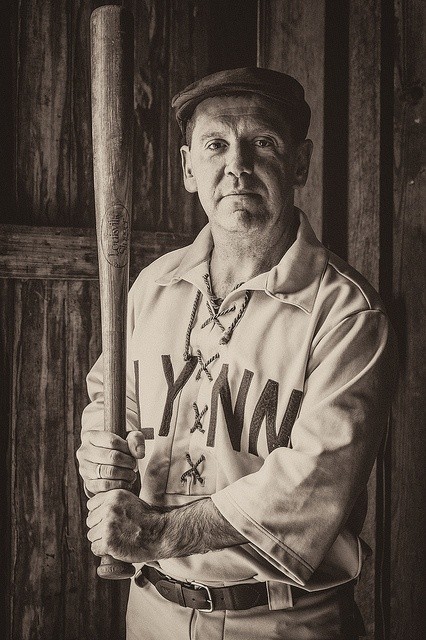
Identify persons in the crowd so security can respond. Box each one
[76,66,400,640]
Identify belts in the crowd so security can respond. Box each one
[140,564,309,612]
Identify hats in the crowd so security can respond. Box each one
[169,66,310,138]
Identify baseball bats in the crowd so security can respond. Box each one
[87,3,134,581]
